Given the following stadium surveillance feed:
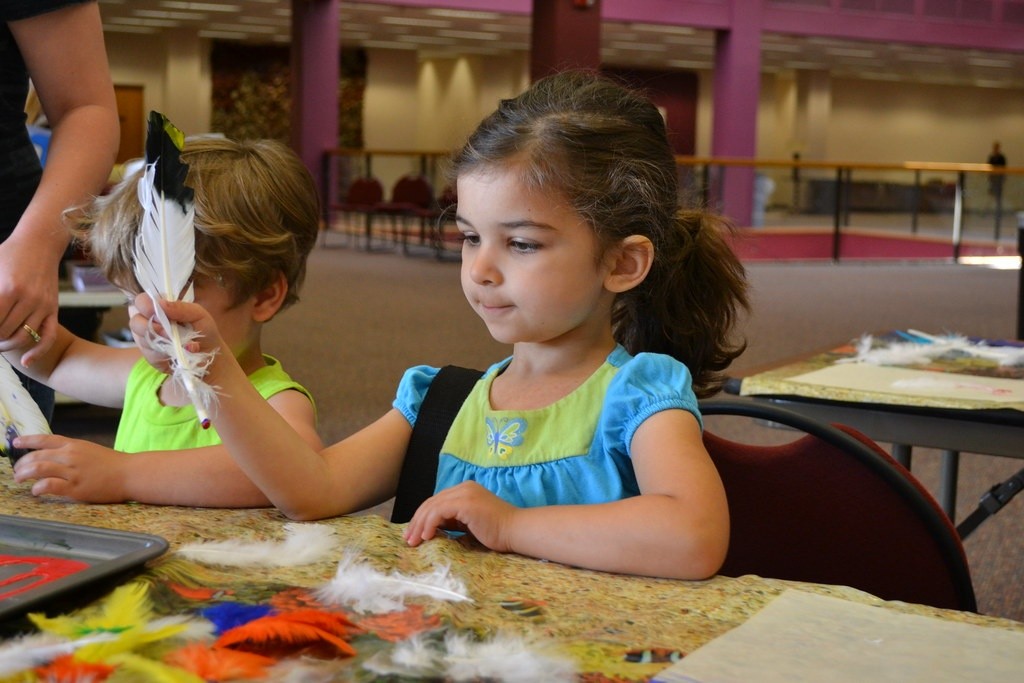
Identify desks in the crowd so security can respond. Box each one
[59,279,129,308]
[722,329,1024,541]
[0,456,1024,683]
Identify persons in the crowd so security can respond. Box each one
[131,66,750,580]
[0,0,120,427]
[987,142,1006,195]
[0,139,321,507]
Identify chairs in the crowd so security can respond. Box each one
[697,399,978,613]
[326,174,458,257]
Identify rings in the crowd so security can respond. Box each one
[24,324,41,343]
